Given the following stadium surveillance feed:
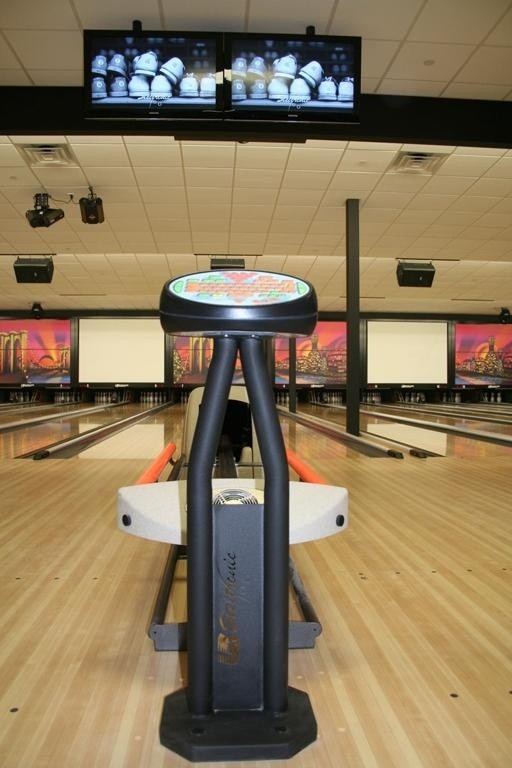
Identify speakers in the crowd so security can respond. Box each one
[14,258,54,283]
[395,263,435,288]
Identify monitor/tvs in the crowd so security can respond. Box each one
[224,30,362,121]
[83,28,224,119]
